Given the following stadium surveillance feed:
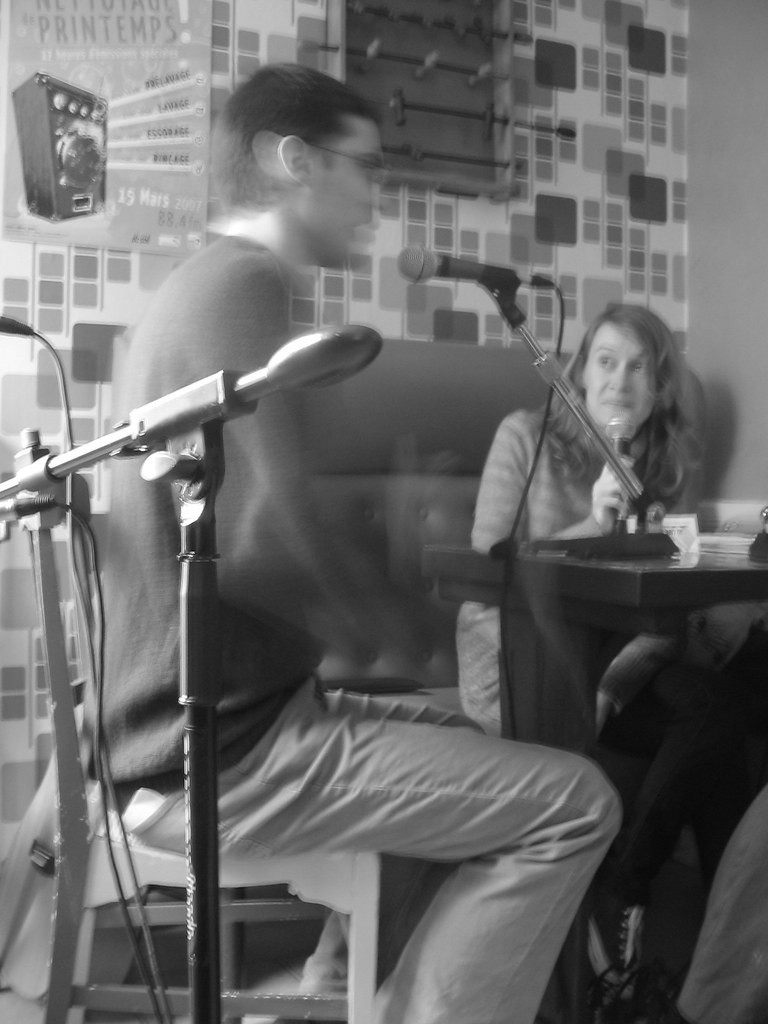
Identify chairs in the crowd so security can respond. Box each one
[21,507,381,1023]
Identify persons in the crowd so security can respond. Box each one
[83,59,620,1022]
[458,304,717,1003]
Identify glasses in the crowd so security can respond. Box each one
[305,144,392,185]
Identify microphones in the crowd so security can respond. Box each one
[604,412,637,533]
[397,245,555,292]
[0,495,56,522]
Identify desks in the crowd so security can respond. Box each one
[416,544,768,1024]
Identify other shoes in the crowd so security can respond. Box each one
[586,903,643,1008]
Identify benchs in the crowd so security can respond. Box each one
[285,329,703,732]
